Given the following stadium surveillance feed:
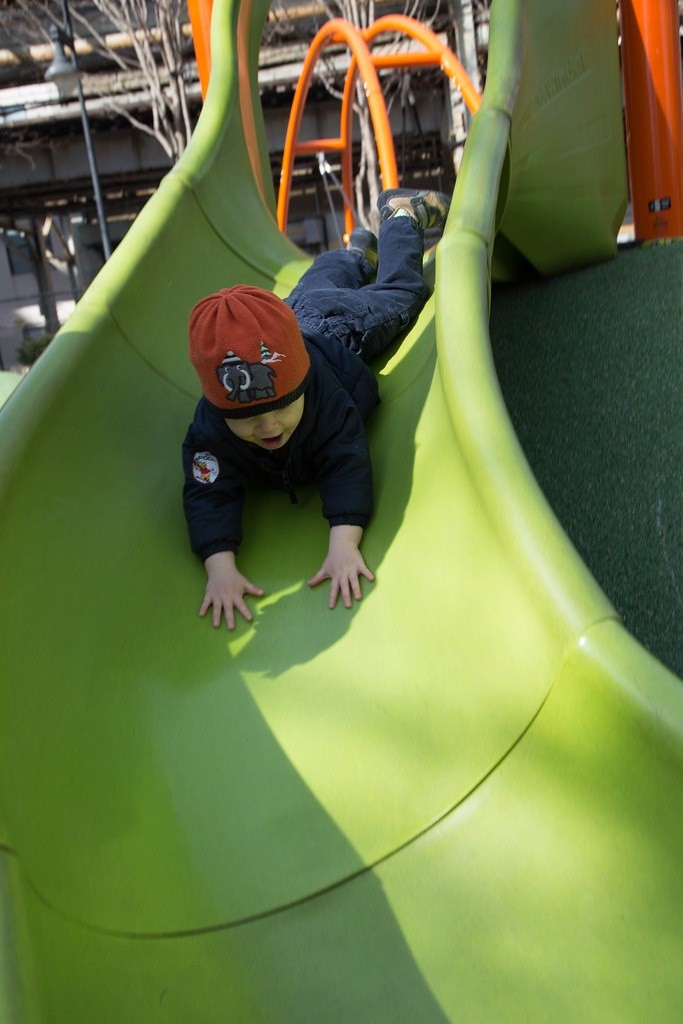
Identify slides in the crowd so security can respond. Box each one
[0,0,681,1022]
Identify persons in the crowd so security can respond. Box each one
[180,185,452,630]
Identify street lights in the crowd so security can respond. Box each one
[44,0,111,267]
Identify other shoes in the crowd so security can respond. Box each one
[379,188,451,232]
[347,229,381,272]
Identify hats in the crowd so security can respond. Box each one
[188,284,309,420]
